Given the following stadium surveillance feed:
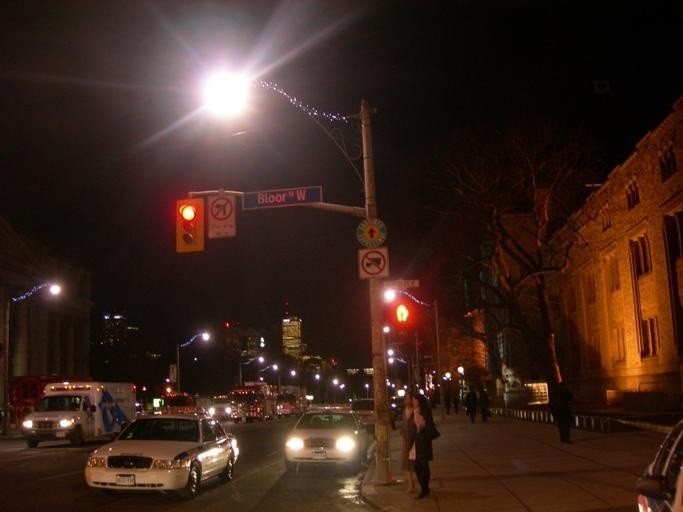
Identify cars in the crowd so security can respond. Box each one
[209,398,231,418]
[635,419,683,512]
[85,415,239,500]
[284,398,374,468]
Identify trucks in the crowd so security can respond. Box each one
[22,382,135,448]
[160,391,210,419]
[231,383,310,424]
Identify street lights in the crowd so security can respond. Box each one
[3,281,62,438]
[385,289,446,408]
[200,70,394,484]
[238,355,264,386]
[175,332,210,393]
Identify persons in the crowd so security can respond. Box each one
[478,389,489,423]
[444,394,450,415]
[452,394,460,414]
[465,387,477,423]
[406,393,436,499]
[547,381,575,444]
[398,392,422,494]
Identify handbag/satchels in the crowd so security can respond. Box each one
[421,422,441,440]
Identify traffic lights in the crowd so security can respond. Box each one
[176,198,205,252]
[394,303,409,327]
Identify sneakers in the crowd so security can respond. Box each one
[414,488,430,499]
[407,486,419,493]
